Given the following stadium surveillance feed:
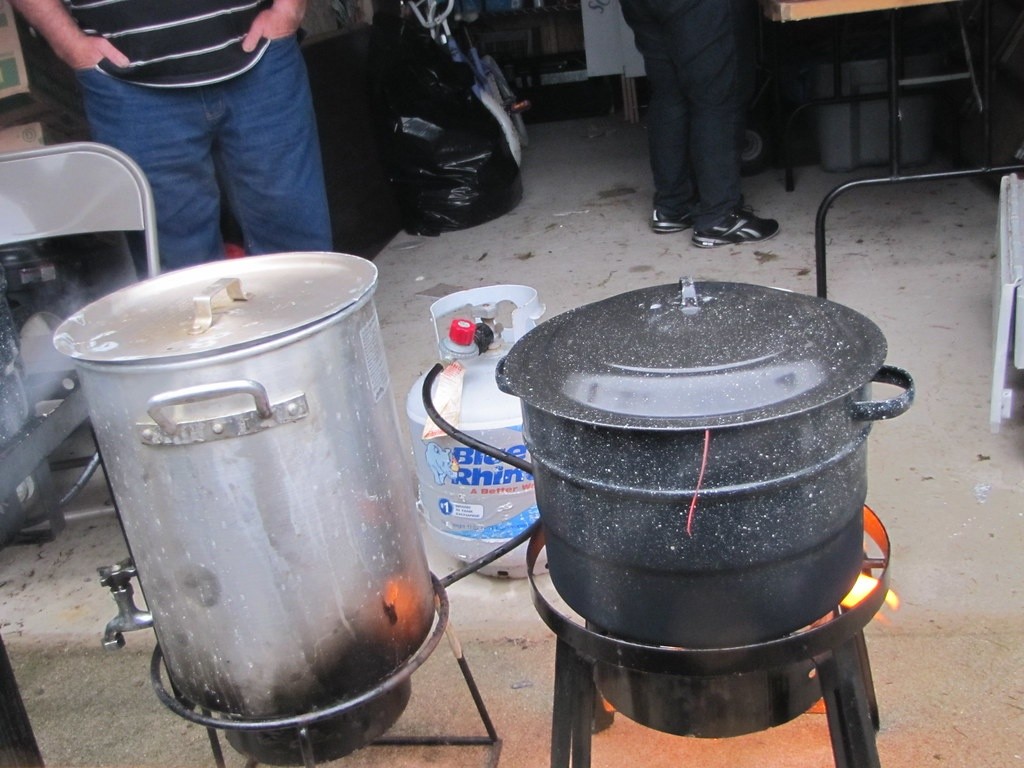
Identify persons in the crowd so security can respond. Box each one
[13,1,334,276]
[619,0,781,249]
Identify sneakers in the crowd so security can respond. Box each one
[651,203,696,233]
[691,196,780,248]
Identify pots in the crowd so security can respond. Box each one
[48,248,433,718]
[494,271,918,654]
